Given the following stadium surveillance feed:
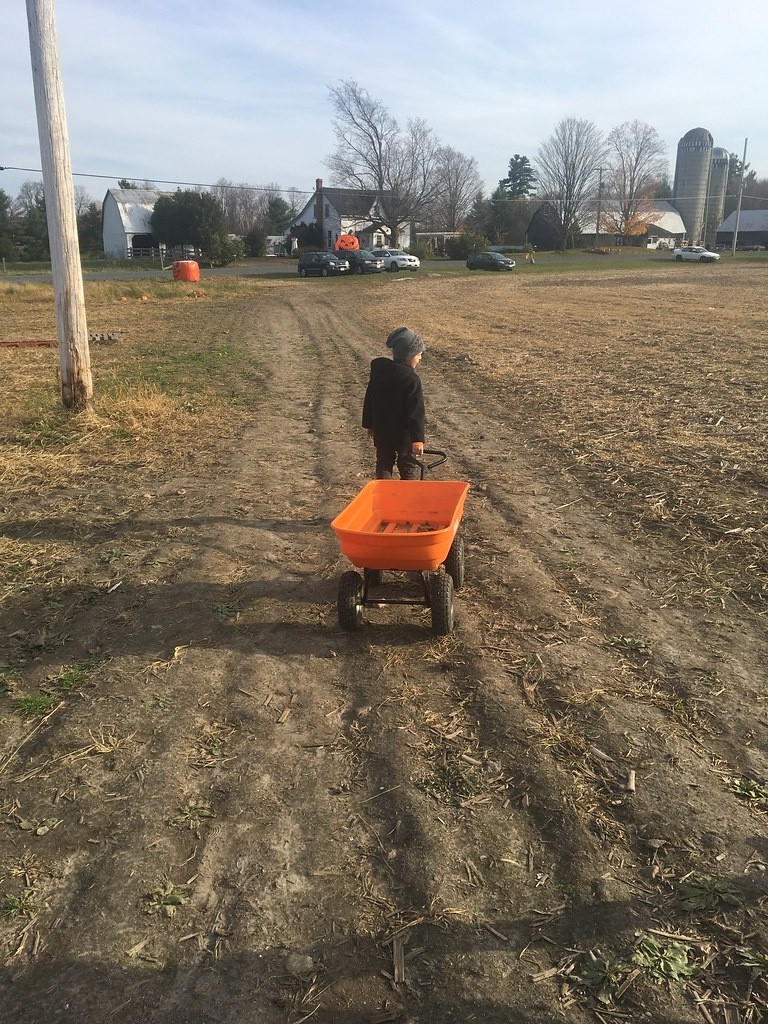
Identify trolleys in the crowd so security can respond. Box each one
[331,448,470,634]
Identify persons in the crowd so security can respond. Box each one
[363,326,425,480]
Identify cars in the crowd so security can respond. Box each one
[466,252,515,271]
[672,246,720,263]
[297,252,350,277]
[332,249,386,274]
[370,249,420,272]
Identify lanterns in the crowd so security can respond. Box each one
[336,230,359,250]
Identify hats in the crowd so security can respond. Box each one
[386,327,427,363]
